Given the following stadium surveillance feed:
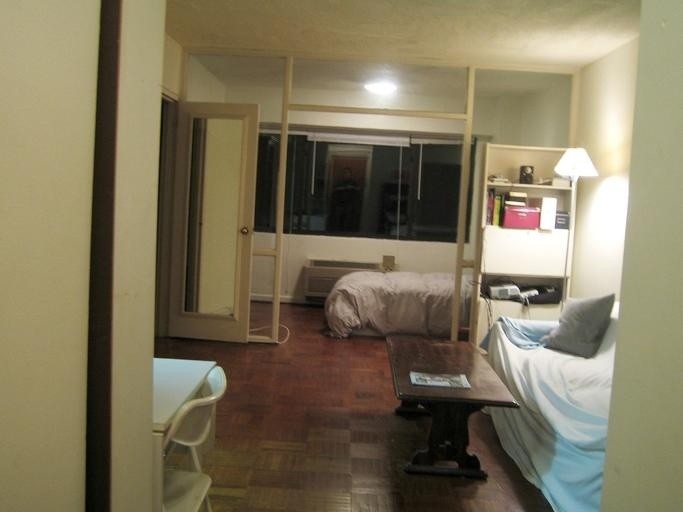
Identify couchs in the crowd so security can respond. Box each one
[489,306,615,512]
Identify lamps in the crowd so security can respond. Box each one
[553,148,599,302]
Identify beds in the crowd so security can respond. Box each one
[325,269,471,338]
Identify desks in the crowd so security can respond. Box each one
[149,357,217,432]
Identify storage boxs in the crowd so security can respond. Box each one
[501,205,541,229]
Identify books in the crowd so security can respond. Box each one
[488,186,526,225]
[325,166,361,233]
[409,370,471,389]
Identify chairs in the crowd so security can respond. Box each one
[151,363,230,512]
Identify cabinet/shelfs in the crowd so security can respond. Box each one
[468,139,576,351]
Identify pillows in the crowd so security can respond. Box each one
[540,291,617,360]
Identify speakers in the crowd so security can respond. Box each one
[519,165,534,184]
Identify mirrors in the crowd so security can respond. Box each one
[252,130,476,248]
